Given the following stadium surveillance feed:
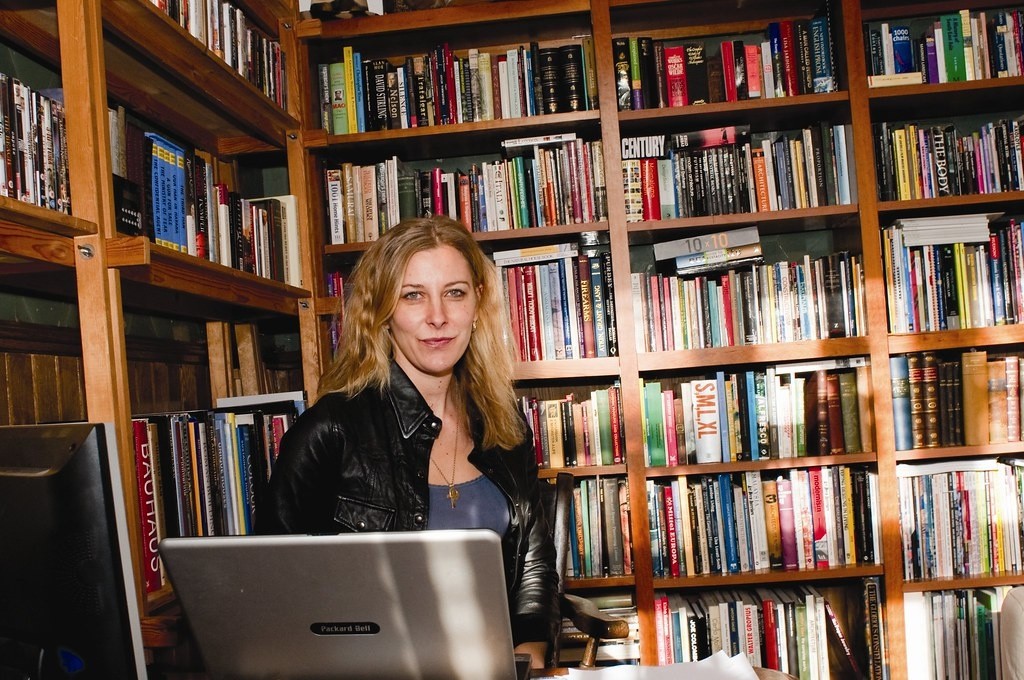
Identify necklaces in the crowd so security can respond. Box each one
[430,408,459,509]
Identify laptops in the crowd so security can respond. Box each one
[158,529,518,680]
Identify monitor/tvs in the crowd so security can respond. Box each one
[0,421,148,680]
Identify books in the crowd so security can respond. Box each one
[0,0,1024,680]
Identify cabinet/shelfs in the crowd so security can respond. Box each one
[0,0,1024,680]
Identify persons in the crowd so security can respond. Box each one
[249,219,556,673]
